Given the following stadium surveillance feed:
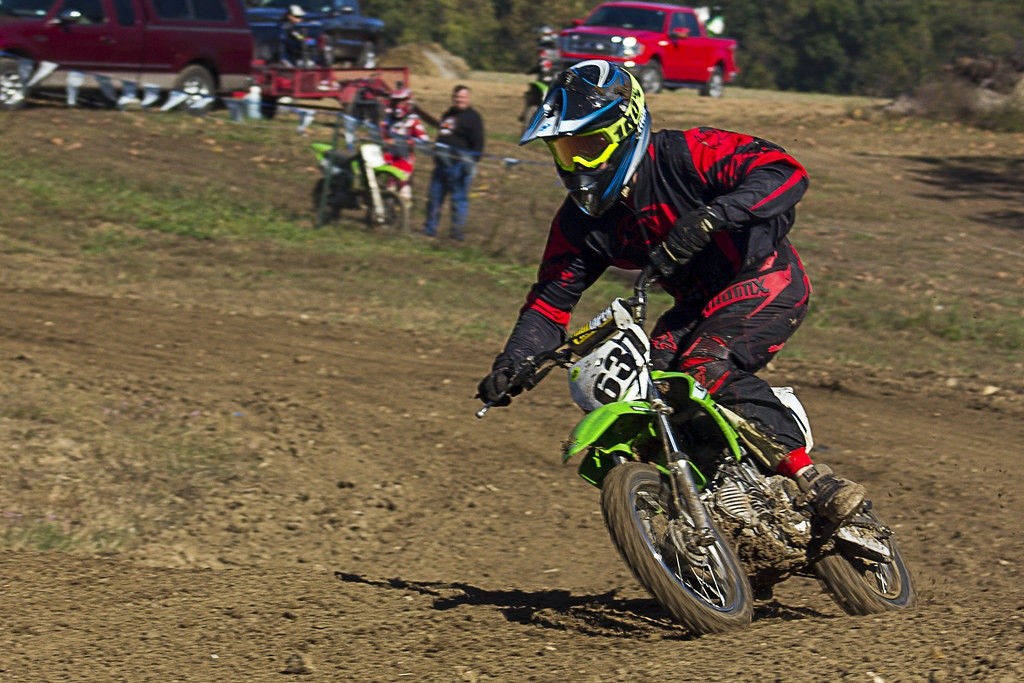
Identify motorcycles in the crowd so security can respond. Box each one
[471,234,913,633]
[309,132,413,236]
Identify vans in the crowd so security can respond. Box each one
[0,1,260,112]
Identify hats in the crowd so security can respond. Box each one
[289,4,306,16]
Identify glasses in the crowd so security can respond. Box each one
[547,127,619,171]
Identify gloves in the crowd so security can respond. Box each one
[477,361,524,406]
[666,205,724,266]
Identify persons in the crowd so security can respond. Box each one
[273,5,318,68]
[367,84,485,244]
[478,59,868,526]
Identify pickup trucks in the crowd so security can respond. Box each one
[539,1,737,99]
[247,0,387,70]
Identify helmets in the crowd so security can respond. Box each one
[389,89,414,119]
[518,60,652,216]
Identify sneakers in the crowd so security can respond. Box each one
[798,464,866,524]
